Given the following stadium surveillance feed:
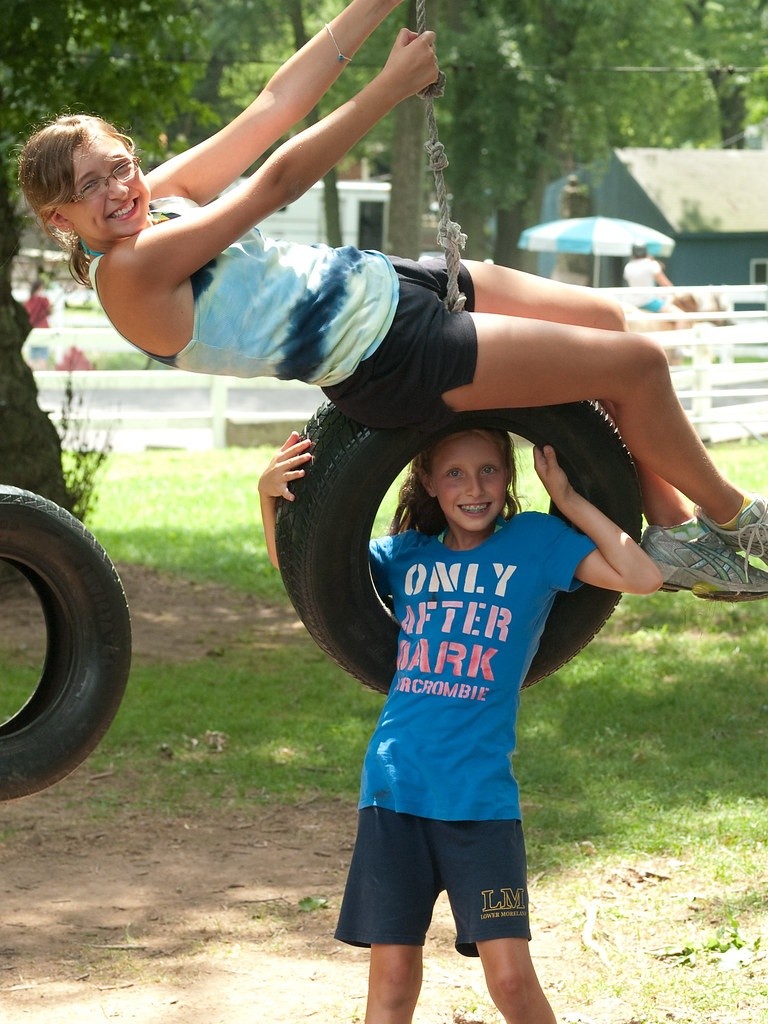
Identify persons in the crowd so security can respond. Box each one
[255,425,663,1024]
[17,0,768,604]
[23,279,53,328]
[624,245,674,288]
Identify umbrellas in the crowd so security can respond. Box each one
[518,216,675,288]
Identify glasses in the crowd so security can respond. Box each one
[65,157,142,207]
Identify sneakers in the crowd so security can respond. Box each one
[693,494,768,584]
[639,516,768,604]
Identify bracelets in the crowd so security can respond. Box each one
[325,24,352,62]
